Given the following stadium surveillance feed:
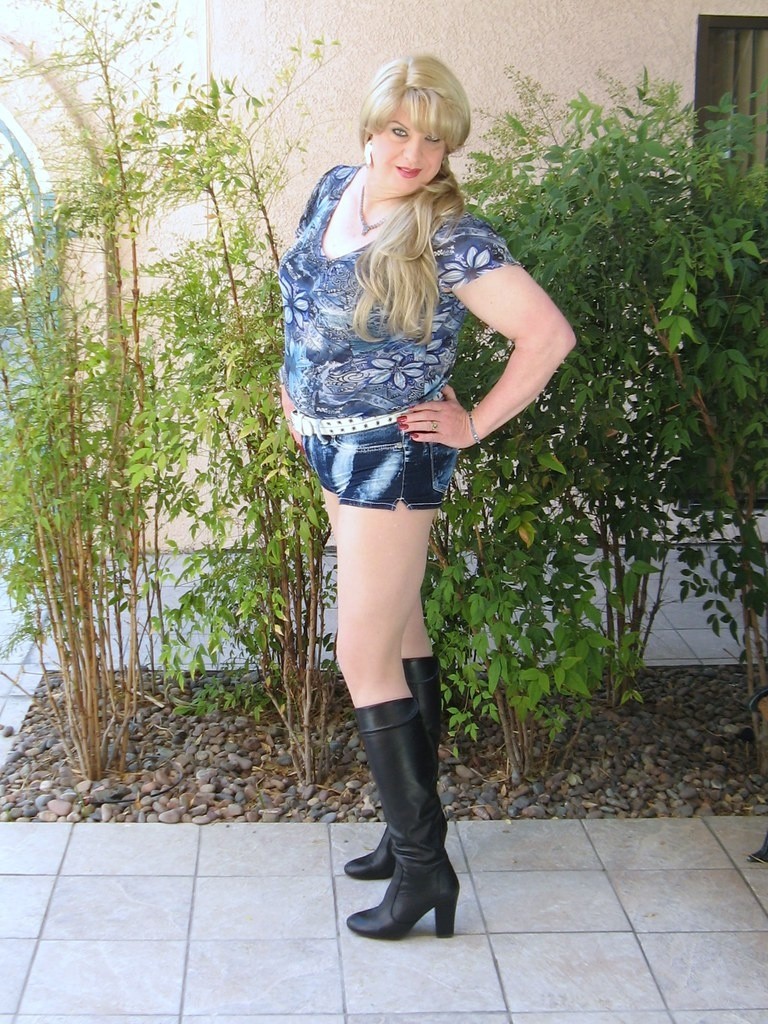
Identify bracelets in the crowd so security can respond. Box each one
[468,410,480,444]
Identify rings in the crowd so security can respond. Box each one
[431,420,438,431]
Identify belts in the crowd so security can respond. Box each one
[290,404,415,436]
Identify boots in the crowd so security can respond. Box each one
[344,654,449,880]
[346,697,460,940]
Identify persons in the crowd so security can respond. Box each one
[280,54,579,942]
